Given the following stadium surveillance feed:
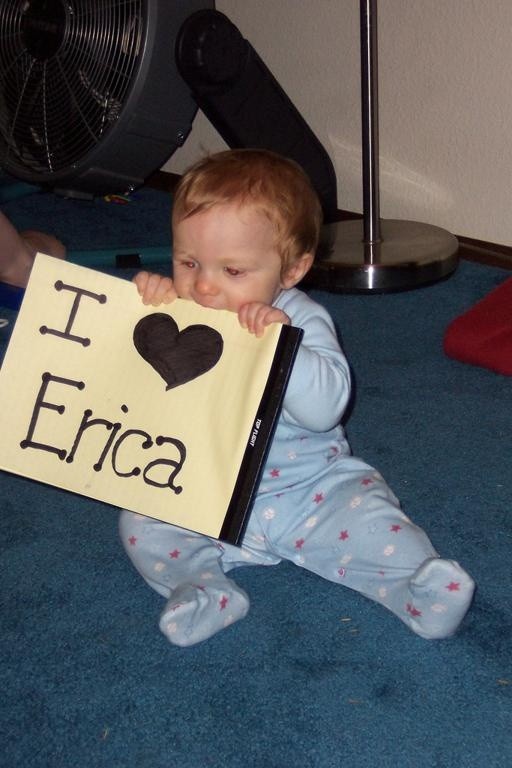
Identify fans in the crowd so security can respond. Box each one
[0,1,335,223]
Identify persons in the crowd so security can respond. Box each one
[0,213,71,367]
[114,148,475,650]
